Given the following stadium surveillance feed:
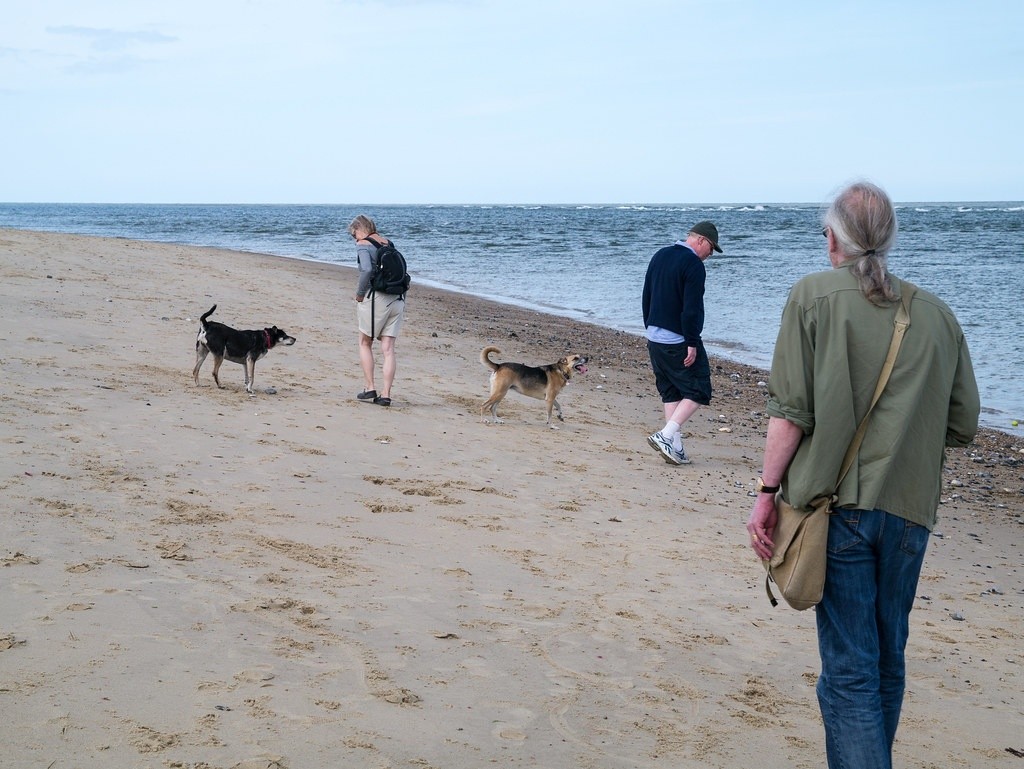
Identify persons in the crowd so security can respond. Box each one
[348,215,405,406]
[642,221,724,465]
[746,181,980,769]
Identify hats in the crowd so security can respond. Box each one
[690,221,723,253]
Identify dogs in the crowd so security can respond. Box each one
[478,345,590,429]
[191,303,296,395]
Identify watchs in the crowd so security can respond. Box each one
[755,477,780,493]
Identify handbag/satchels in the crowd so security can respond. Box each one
[759,493,831,611]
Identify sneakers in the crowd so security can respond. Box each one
[646,431,681,465]
[674,445,692,463]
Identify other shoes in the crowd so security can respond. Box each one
[357,388,377,402]
[373,395,391,406]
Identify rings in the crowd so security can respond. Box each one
[751,535,757,541]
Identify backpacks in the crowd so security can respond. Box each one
[357,237,411,295]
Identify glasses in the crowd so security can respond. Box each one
[822,226,831,237]
[352,231,358,239]
